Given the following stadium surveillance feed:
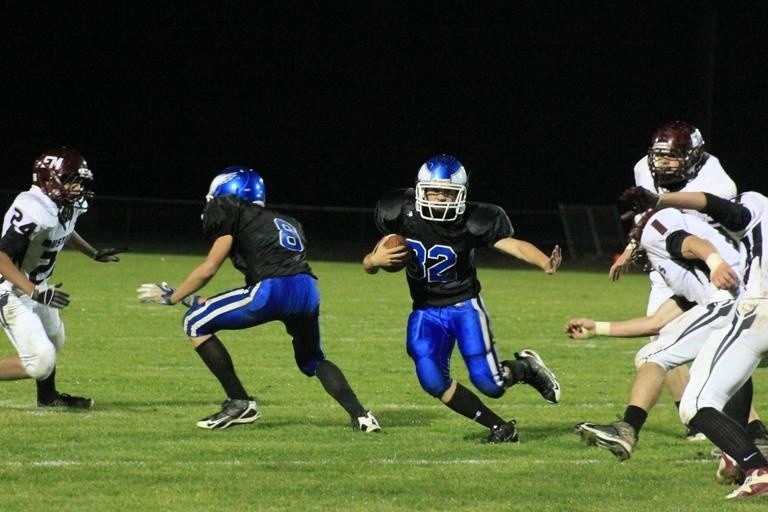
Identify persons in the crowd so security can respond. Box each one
[363,155,563,445]
[616,186,768,501]
[562,186,753,486]
[608,117,763,448]
[136,165,381,433]
[0,143,131,411]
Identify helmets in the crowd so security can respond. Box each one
[31,144,97,210]
[205,164,268,208]
[646,120,709,191]
[416,153,469,222]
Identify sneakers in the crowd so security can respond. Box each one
[722,466,768,502]
[573,418,638,461]
[756,441,768,460]
[31,392,94,412]
[488,422,521,447]
[517,347,562,404]
[353,410,381,434]
[195,397,263,429]
[717,452,741,487]
[687,429,706,443]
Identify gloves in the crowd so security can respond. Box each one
[617,183,658,219]
[93,245,120,263]
[31,280,70,311]
[136,280,176,307]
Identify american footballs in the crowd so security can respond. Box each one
[374,234,410,273]
